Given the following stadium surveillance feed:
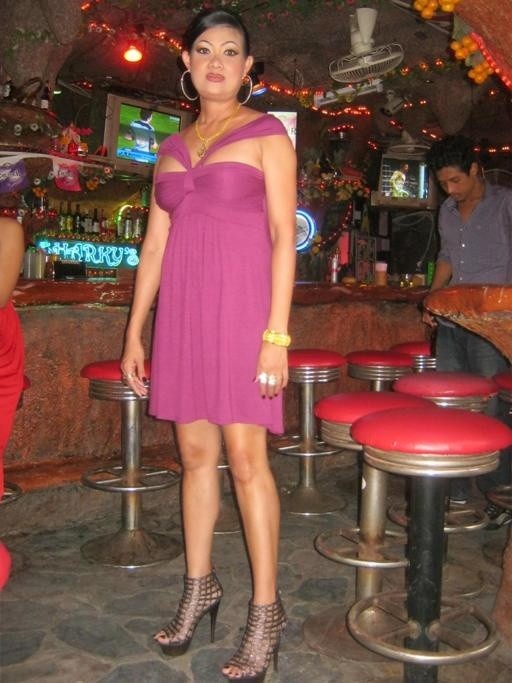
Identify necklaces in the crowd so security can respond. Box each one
[194,102,241,160]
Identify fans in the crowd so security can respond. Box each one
[327,5,405,84]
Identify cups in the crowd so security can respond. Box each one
[374,263,387,286]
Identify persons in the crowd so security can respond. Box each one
[122,107,158,162]
[387,162,410,199]
[418,138,510,531]
[0,213,31,589]
[116,5,305,682]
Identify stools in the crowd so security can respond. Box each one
[76,359,187,570]
[174,453,248,535]
[0,375,34,506]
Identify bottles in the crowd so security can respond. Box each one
[100,208,106,235]
[36,249,46,279]
[109,209,117,235]
[74,204,81,231]
[66,200,74,232]
[24,244,36,279]
[116,211,124,237]
[124,209,133,240]
[133,209,142,244]
[92,207,100,233]
[58,200,66,231]
[81,208,92,233]
[17,195,57,223]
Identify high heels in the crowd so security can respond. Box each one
[224,590,286,683]
[156,570,223,656]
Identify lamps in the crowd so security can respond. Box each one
[121,44,144,63]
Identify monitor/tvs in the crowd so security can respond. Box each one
[265,106,303,168]
[86,90,190,183]
[370,152,439,212]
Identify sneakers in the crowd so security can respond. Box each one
[480,503,511,532]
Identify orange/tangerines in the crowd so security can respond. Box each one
[450,36,479,60]
[412,0,459,20]
[467,61,494,85]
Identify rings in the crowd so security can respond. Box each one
[257,373,267,384]
[124,372,134,378]
[269,374,276,385]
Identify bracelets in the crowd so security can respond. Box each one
[261,330,296,348]
